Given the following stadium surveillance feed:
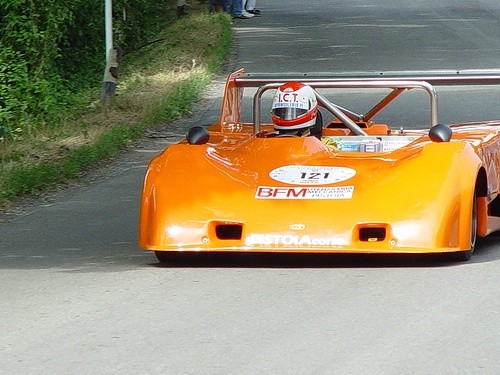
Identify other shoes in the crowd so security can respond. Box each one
[234,10,260,19]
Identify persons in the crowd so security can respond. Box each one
[224,0,261,19]
[267,82,316,137]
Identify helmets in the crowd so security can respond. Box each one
[270,81,318,130]
